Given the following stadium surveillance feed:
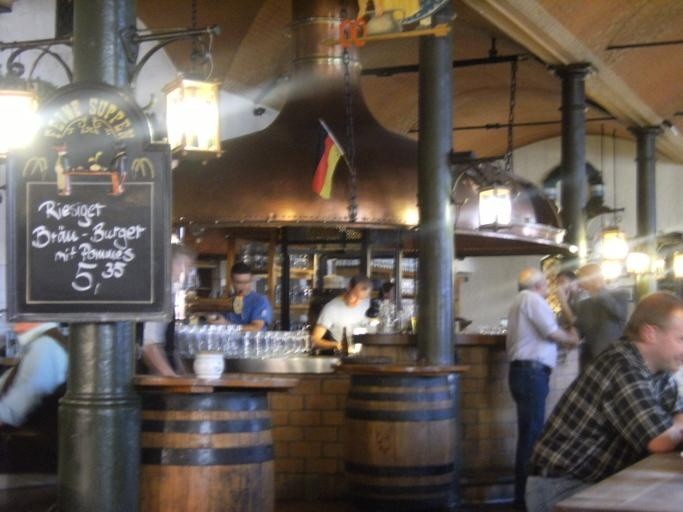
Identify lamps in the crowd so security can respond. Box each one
[587,196,629,259]
[1,37,72,160]
[450,149,515,230]
[126,27,227,166]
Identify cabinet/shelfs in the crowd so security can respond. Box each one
[189,241,420,332]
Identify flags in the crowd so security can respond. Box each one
[311,132,345,202]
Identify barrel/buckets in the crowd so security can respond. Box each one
[139,389,276,511]
[341,371,462,511]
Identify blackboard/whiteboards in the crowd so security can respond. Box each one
[6,142,174,324]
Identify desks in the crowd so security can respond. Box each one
[554,448,683,510]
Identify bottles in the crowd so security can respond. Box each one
[174,322,316,360]
[239,244,313,307]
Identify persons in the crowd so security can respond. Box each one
[505,266,584,511]
[364,282,397,320]
[309,272,378,358]
[522,289,683,512]
[192,261,273,333]
[548,269,590,324]
[0,319,70,479]
[134,241,191,376]
[572,262,630,378]
